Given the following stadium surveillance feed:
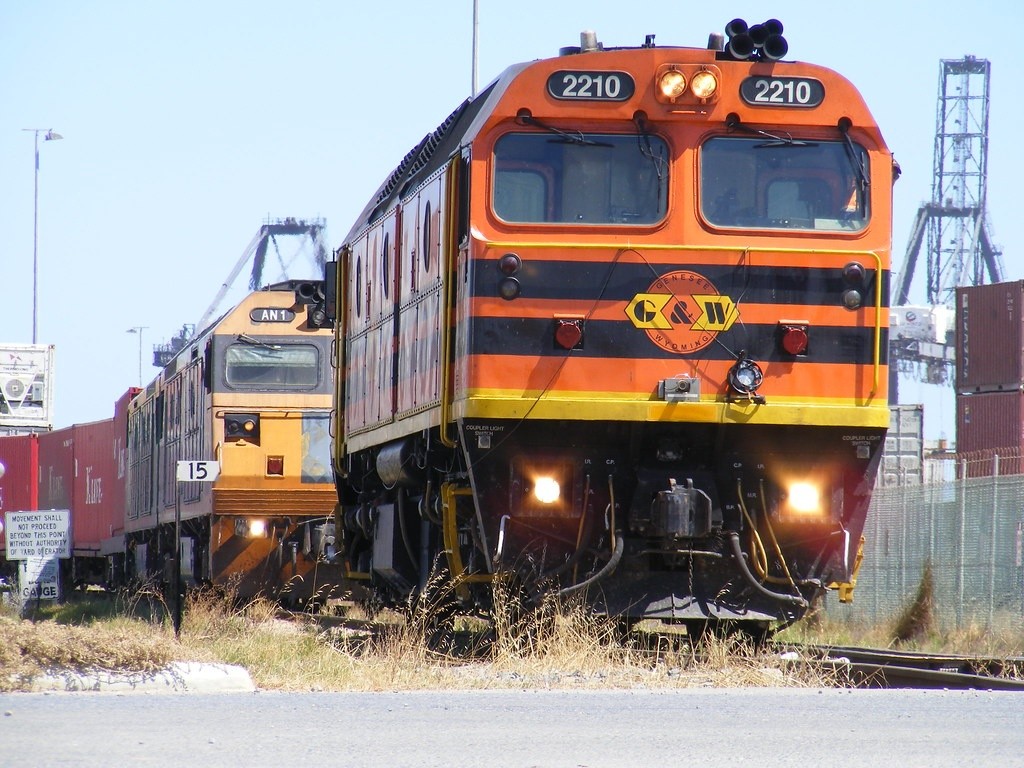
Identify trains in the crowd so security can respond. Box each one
[334,16,900,635]
[0,279,336,605]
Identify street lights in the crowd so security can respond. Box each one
[127,326,149,388]
[20,127,64,343]
